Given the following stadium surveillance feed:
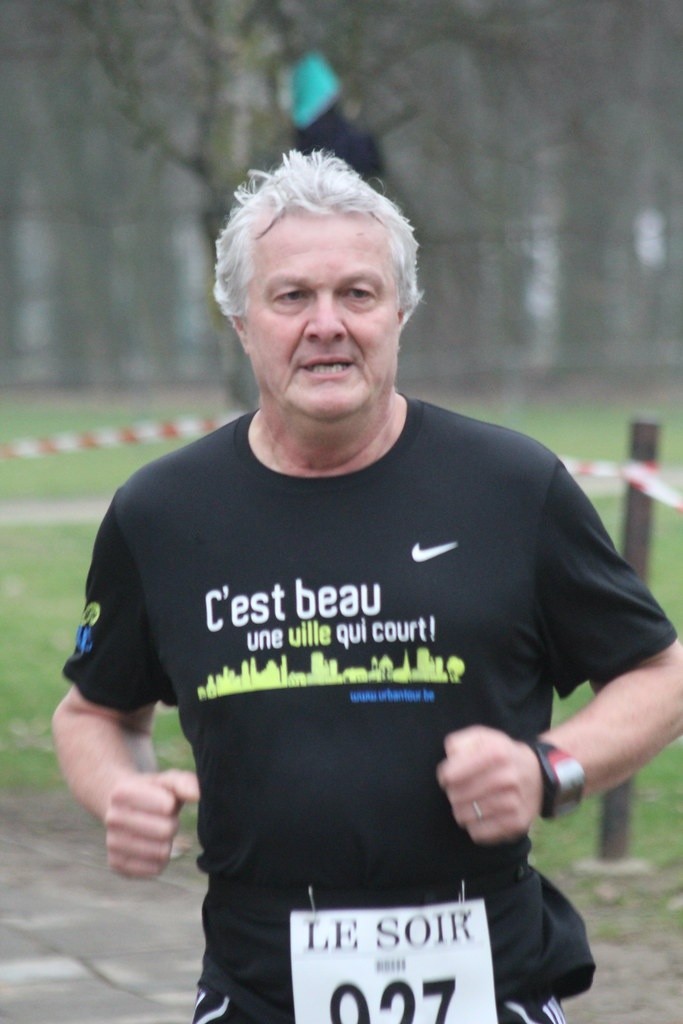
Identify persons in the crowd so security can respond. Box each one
[52,150,682,1024]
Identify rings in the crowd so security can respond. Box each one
[471,801,486,823]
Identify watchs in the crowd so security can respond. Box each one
[531,740,584,820]
